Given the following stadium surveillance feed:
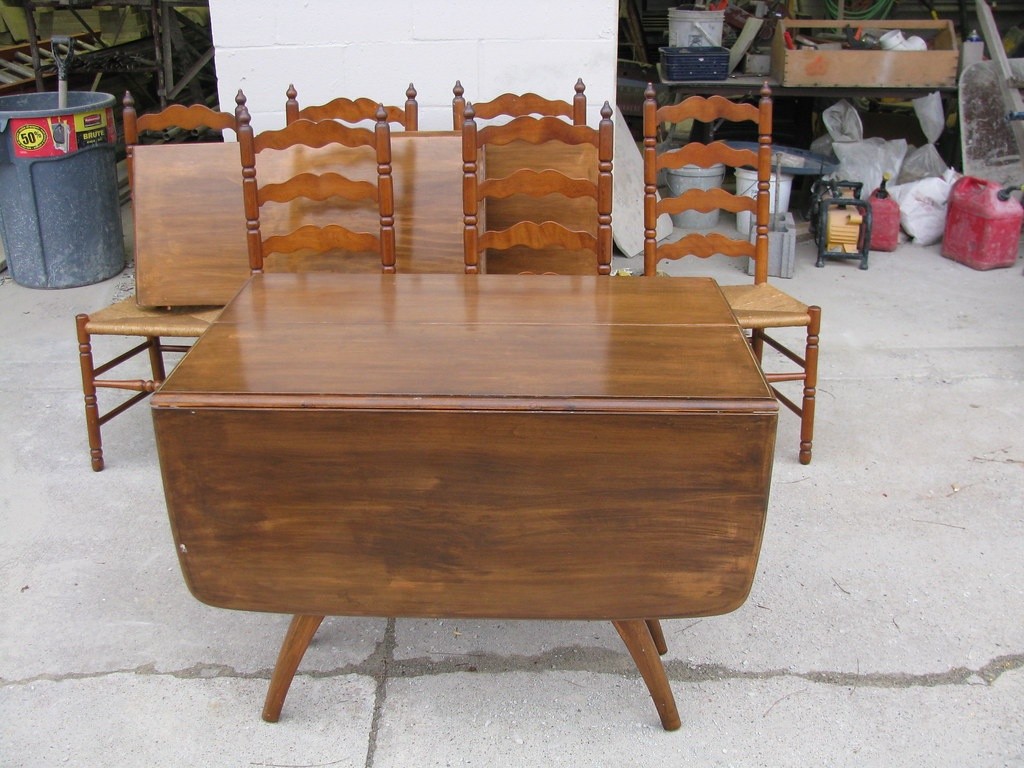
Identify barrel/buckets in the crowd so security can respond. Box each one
[940,175,1024,273]
[667,6,725,48]
[0,92,127,291]
[662,147,726,230]
[734,166,795,236]
[859,173,902,253]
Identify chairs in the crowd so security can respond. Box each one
[75,78,821,471]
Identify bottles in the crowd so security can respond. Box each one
[965,29,982,42]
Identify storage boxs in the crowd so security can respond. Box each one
[658,46,732,81]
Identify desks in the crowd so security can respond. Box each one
[149,269,779,729]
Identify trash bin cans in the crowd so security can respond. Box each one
[0,91,127,289]
